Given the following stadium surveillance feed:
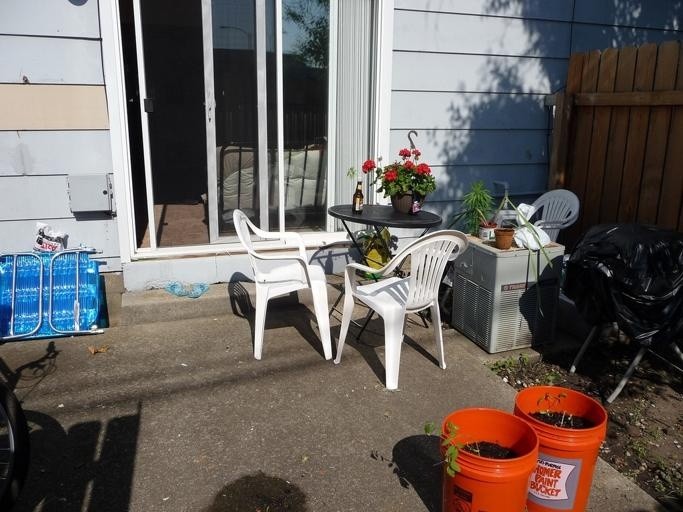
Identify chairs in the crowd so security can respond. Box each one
[333,229,468,391]
[494,189,579,242]
[233,209,333,361]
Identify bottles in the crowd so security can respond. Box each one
[353,181,364,215]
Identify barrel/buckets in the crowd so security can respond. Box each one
[440,407,540,512]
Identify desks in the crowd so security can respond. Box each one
[328,205,443,340]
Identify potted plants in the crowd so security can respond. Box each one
[424,409,539,512]
[463,183,497,240]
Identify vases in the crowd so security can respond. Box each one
[391,194,426,214]
[494,229,515,249]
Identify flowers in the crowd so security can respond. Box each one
[362,149,437,199]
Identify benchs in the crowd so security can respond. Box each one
[0,247,104,342]
[200,136,326,222]
